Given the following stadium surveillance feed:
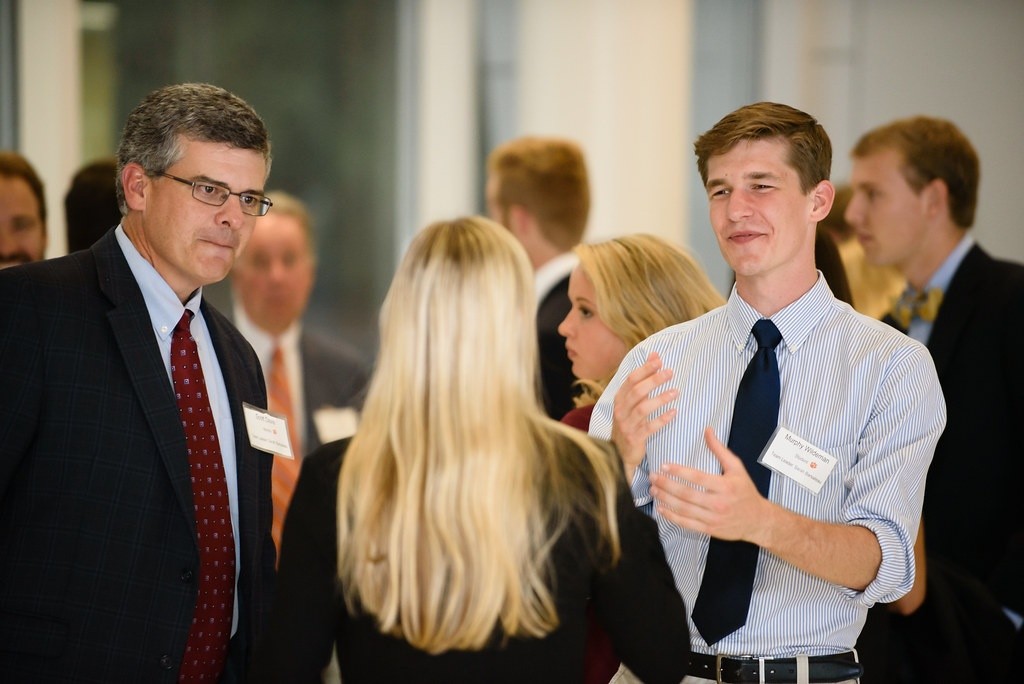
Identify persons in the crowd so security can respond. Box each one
[588,103,946,684]
[558,234,726,432]
[217,193,370,576]
[488,137,594,420]
[815,118,1024,684]
[273,214,691,684]
[0,83,278,684]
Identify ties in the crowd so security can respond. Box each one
[170,311,235,684]
[691,318,783,646]
[266,348,300,570]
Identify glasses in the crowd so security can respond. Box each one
[147,170,274,216]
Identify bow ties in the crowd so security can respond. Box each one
[887,288,943,330]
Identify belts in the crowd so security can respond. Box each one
[686,650,863,684]
